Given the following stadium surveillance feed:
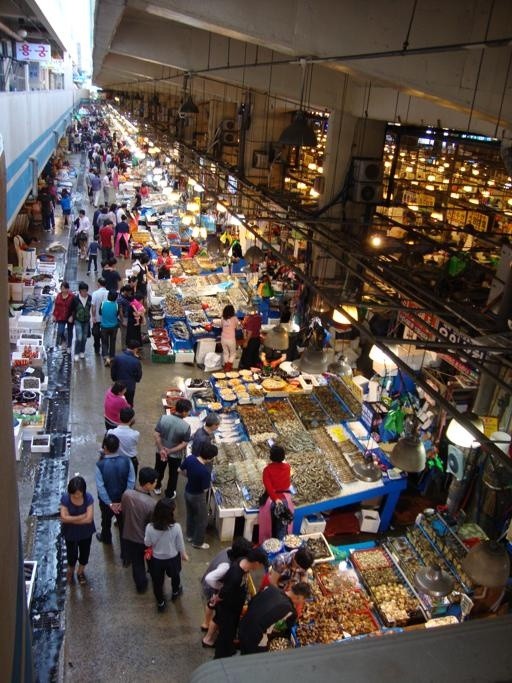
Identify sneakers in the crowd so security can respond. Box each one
[186,536,194,542]
[80,352,85,358]
[155,486,163,494]
[104,357,111,366]
[191,541,210,549]
[167,490,178,499]
[157,593,167,613]
[171,584,183,601]
[74,354,79,361]
[68,577,76,588]
[134,576,150,595]
[78,570,87,587]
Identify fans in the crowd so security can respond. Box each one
[445,445,466,481]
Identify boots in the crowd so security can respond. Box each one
[223,361,233,372]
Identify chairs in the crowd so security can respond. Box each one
[13,234,37,268]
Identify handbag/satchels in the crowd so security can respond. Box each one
[142,546,154,560]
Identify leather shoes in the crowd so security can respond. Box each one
[95,531,114,544]
[121,557,132,567]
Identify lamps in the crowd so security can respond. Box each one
[445,353,487,449]
[414,448,512,595]
[18,18,29,40]
[109,71,199,114]
[368,310,401,365]
[373,360,398,377]
[332,303,358,325]
[390,368,428,473]
[278,66,318,146]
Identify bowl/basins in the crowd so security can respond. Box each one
[208,402,222,413]
[152,327,171,355]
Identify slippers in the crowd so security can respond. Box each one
[201,624,209,631]
[200,639,218,648]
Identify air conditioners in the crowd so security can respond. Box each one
[254,152,269,169]
[222,119,240,132]
[352,159,383,182]
[222,133,238,145]
[350,182,385,202]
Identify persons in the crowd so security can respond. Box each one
[96,381,221,613]
[200,537,313,660]
[112,341,142,406]
[260,446,291,542]
[59,476,97,587]
[220,255,322,373]
[40,109,172,366]
[188,237,199,258]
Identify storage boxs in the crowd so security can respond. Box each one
[299,513,335,567]
[8,261,56,461]
[358,509,381,534]
[23,560,38,614]
[114,182,216,364]
